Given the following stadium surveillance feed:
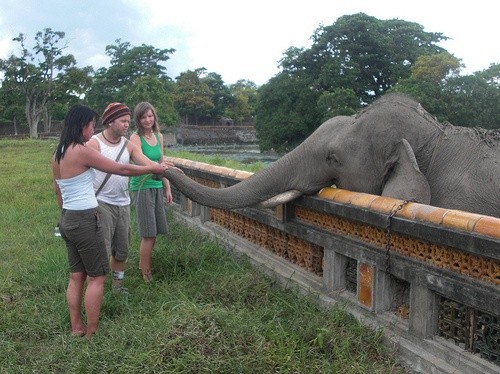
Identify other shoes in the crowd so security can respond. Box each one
[112,287,129,294]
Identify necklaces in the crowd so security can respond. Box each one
[143,132,153,140]
[101,131,122,144]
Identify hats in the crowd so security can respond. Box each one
[100,101,131,124]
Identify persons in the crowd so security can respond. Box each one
[85,102,175,297]
[52,105,172,338]
[128,102,172,287]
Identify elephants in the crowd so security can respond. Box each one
[152,90,500,218]
